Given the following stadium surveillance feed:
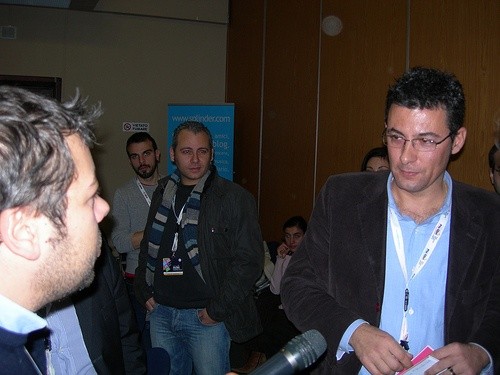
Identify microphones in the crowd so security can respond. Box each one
[248,329,327,375]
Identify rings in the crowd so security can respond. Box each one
[447,367,456,375]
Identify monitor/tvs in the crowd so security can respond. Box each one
[0,75,62,103]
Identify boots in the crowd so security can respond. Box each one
[230,351,267,375]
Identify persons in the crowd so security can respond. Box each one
[280,66,500,375]
[0,86,110,375]
[34,248,147,375]
[135,120,265,375]
[111,132,165,348]
[231,216,308,375]
[389,207,450,375]
[360,147,390,172]
[488,143,500,195]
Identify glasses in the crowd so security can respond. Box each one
[380,128,453,151]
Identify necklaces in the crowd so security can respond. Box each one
[135,176,161,207]
